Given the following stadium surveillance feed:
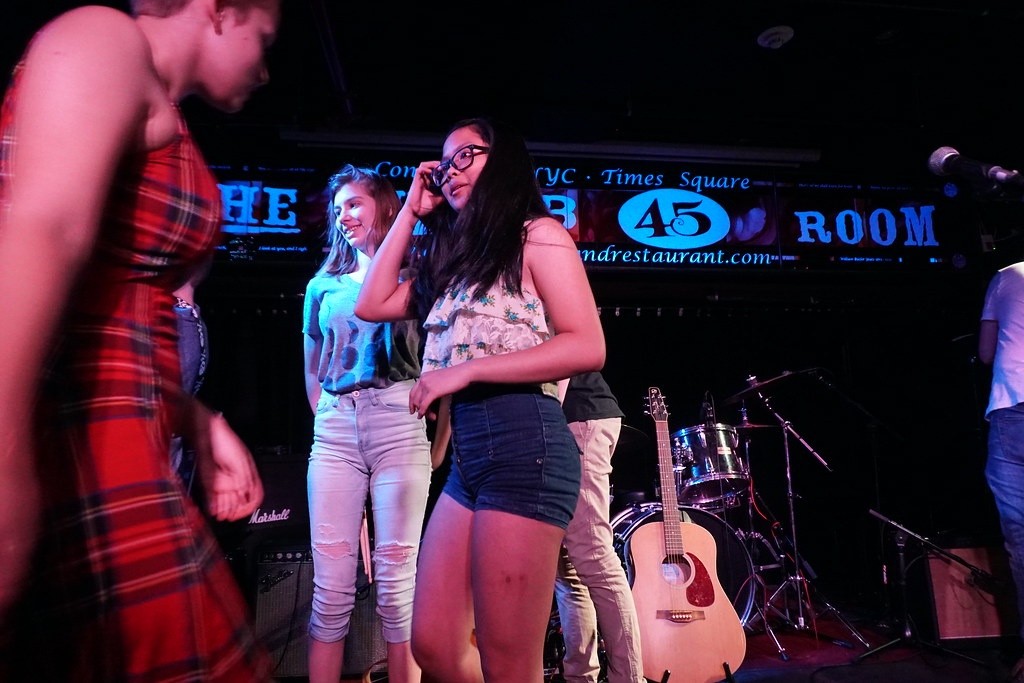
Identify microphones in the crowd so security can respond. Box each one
[700,393,713,422]
[966,572,998,593]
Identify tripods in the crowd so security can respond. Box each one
[744,390,1018,674]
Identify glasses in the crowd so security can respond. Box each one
[429,144,490,188]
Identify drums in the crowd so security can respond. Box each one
[610,501,755,630]
[671,421,749,507]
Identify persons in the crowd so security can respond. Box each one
[354,115,606,683]
[0,0,281,683]
[300,164,452,683]
[170,249,210,474]
[557,371,647,683]
[979,258,1024,643]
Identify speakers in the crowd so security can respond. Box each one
[255,545,391,678]
[918,536,1021,642]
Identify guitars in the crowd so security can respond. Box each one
[623,383,746,683]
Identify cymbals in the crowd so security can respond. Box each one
[618,422,649,448]
[732,368,792,401]
[730,422,781,432]
